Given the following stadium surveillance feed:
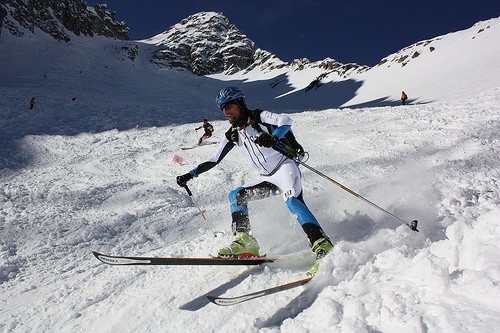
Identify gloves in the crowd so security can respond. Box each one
[176,173,192,186]
[256,132,278,149]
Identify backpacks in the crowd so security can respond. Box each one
[253,108,305,159]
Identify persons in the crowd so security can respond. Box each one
[195,119,214,146]
[177,87,334,277]
[30,97,35,109]
[401,91,405,104]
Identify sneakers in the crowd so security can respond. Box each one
[305,238,335,278]
[218,231,260,257]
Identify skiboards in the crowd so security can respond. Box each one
[181,141,217,150]
[91,250,315,307]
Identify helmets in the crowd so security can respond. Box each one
[215,85,246,113]
